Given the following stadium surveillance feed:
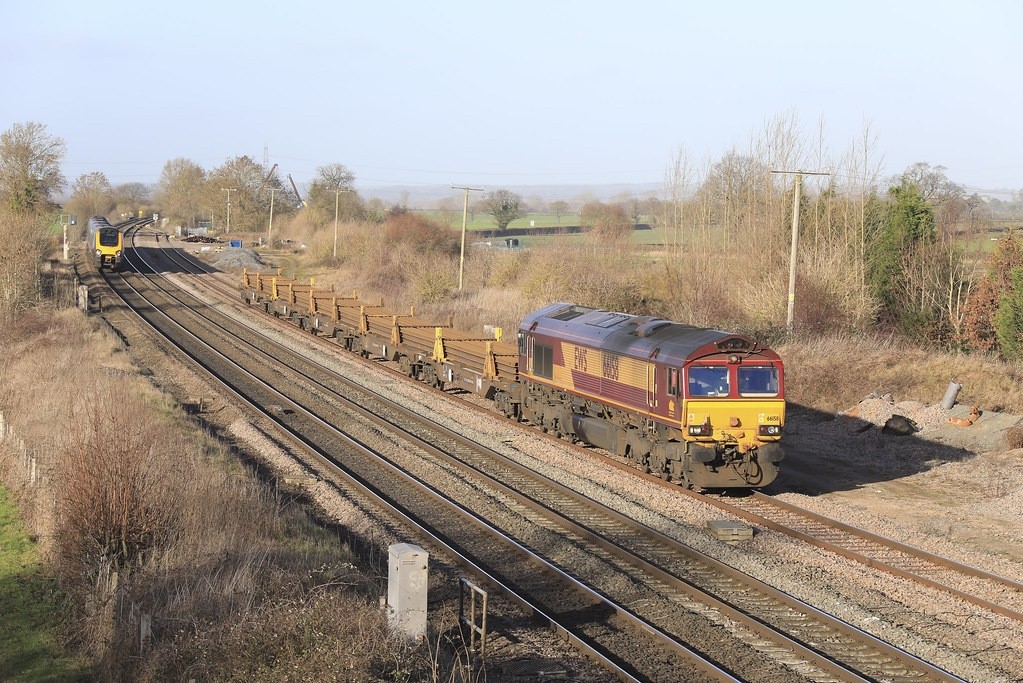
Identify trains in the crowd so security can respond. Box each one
[237,268,785,496]
[87,215,125,272]
